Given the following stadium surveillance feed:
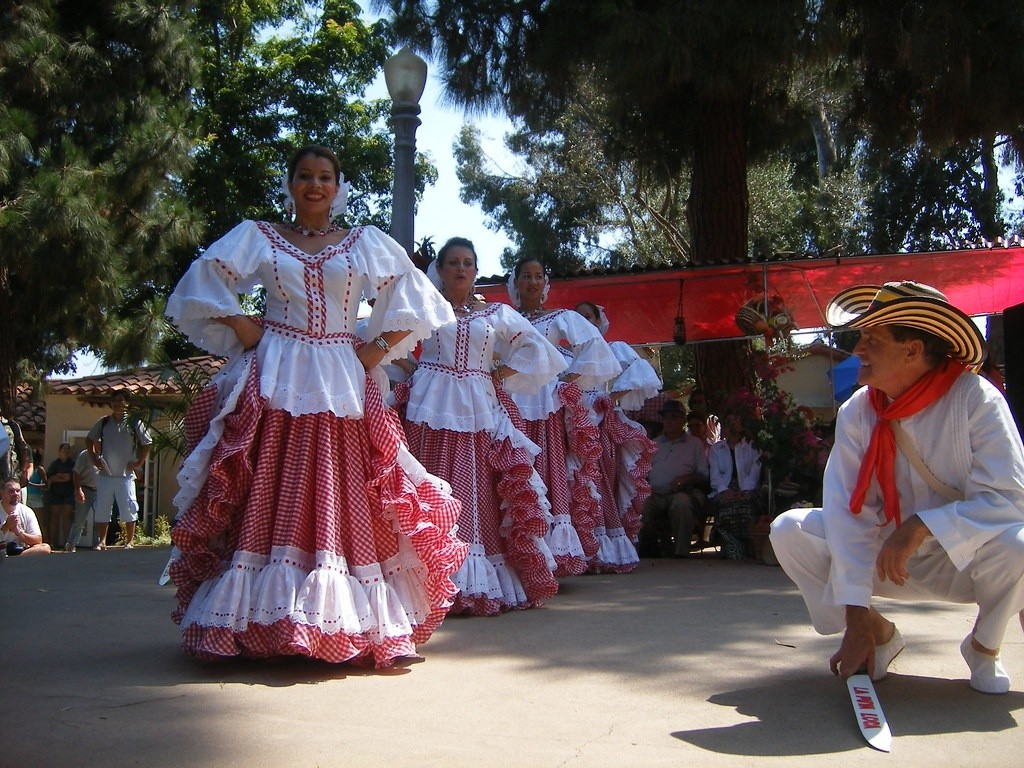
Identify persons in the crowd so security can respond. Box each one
[0,478,51,556]
[628,393,770,559]
[384,237,568,620]
[66,440,101,552]
[489,257,622,575]
[0,412,48,544]
[84,390,152,551]
[169,143,468,669]
[982,349,1004,391]
[575,302,663,575]
[768,279,1024,694]
[46,443,74,551]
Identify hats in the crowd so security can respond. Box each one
[657,400,686,416]
[826,280,988,365]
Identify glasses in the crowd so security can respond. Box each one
[692,399,706,406]
[687,422,705,429]
[110,397,125,404]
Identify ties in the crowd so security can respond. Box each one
[849,355,964,529]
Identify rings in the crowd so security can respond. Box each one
[678,482,680,485]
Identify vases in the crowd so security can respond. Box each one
[751,515,779,565]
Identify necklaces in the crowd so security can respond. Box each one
[442,293,477,313]
[284,225,339,237]
[531,305,544,316]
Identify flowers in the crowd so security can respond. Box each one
[721,388,833,475]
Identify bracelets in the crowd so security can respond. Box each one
[405,364,415,374]
[497,368,503,380]
[374,336,390,353]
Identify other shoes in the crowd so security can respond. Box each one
[123,542,135,550]
[93,542,107,551]
[960,633,1010,693]
[64,545,76,553]
[873,621,906,679]
